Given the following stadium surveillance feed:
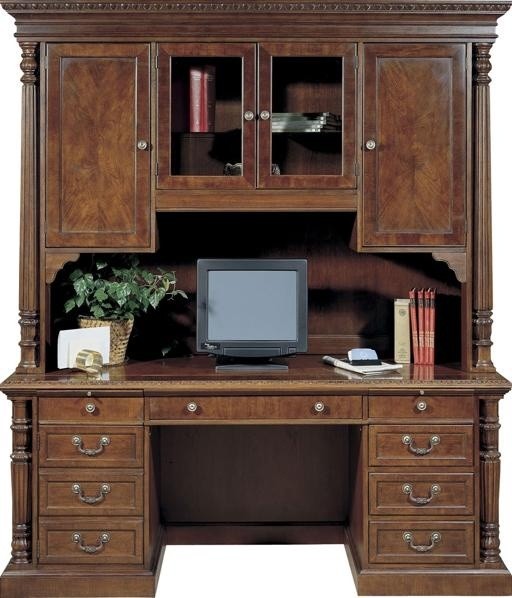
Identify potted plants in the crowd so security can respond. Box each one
[60,255,191,368]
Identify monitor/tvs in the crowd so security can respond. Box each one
[196,258,307,370]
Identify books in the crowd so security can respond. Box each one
[321,355,404,375]
[188,62,216,134]
[271,109,340,134]
[392,286,437,365]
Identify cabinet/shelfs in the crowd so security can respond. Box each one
[0,0,512,598]
